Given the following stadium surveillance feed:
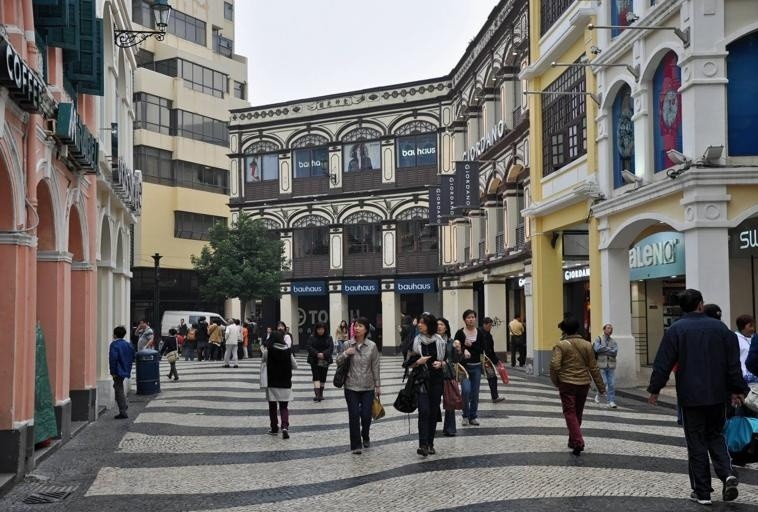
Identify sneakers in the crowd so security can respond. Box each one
[606,401,618,409]
[720,476,739,503]
[414,444,437,457]
[492,397,506,404]
[593,394,603,407]
[112,411,130,420]
[688,490,714,506]
[567,440,586,457]
[266,424,280,435]
[351,436,370,454]
[460,414,482,426]
[280,424,290,439]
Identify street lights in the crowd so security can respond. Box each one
[151,251,165,352]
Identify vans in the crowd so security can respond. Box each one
[161,310,228,342]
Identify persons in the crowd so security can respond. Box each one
[305,322,334,402]
[550,312,618,455]
[508,313,527,368]
[110,326,136,419]
[130,312,263,381]
[259,321,297,438]
[399,309,506,456]
[334,315,381,455]
[646,289,758,503]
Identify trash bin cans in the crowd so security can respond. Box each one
[136,349,161,394]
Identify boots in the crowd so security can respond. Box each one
[313,386,326,402]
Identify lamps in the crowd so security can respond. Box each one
[702,143,724,163]
[114,0,173,48]
[590,46,601,54]
[620,170,642,183]
[320,153,336,185]
[625,12,639,24]
[665,149,692,164]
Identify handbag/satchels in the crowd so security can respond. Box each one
[392,368,422,414]
[333,351,351,388]
[441,361,464,412]
[370,391,386,421]
[723,393,758,464]
[480,349,499,381]
[258,349,271,393]
[164,350,180,363]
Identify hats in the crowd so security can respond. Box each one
[557,317,586,336]
[264,330,287,345]
